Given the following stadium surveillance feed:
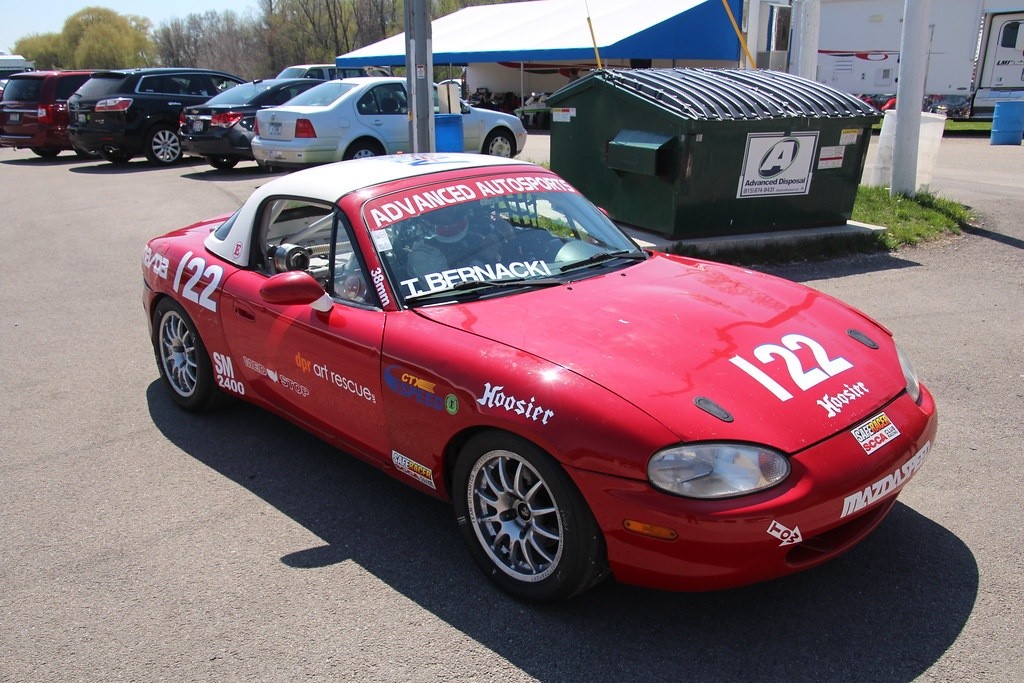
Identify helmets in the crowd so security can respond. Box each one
[417,203,468,243]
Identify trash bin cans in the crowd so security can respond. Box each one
[539,66,887,241]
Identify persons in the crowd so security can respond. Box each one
[407,205,501,292]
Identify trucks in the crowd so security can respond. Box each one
[0,55,36,103]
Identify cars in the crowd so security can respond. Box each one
[0,70,95,159]
[925,99,968,118]
[251,77,528,172]
[177,79,329,169]
[275,64,367,81]
[67,67,248,165]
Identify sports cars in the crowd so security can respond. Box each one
[141,153,939,609]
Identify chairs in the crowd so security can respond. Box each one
[361,92,373,104]
[188,78,206,91]
[391,216,423,265]
[381,98,399,113]
[276,89,291,103]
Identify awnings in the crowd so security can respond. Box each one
[336,0,743,108]
